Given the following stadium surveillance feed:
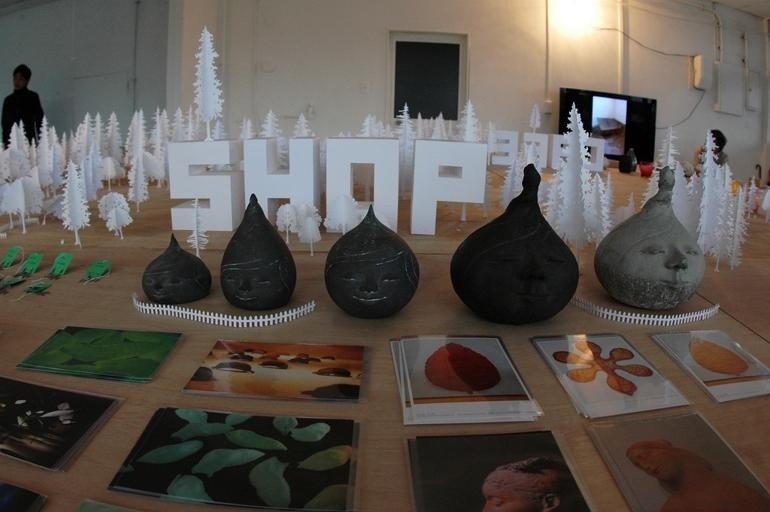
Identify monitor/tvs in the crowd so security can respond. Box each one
[559,88,657,176]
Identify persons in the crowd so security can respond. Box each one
[694,127,729,177]
[626,438,770,512]
[40,402,75,437]
[2,64,43,150]
[480,456,590,512]
